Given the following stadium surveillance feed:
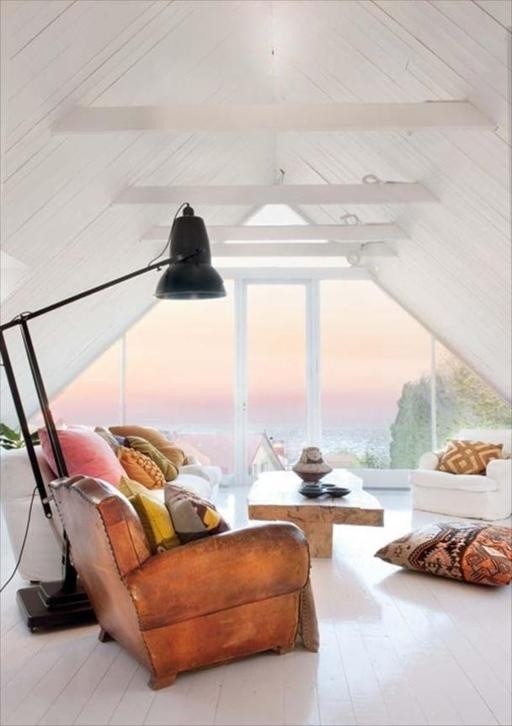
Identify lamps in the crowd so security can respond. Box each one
[1,203,226,634]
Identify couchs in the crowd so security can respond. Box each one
[0,424,223,584]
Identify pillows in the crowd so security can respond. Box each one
[374,520,511,587]
[94,426,121,456]
[436,436,503,475]
[164,483,231,545]
[124,435,179,481]
[118,474,181,558]
[36,427,130,491]
[109,425,190,469]
[117,446,167,490]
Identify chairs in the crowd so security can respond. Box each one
[408,426,512,523]
[48,470,320,690]
